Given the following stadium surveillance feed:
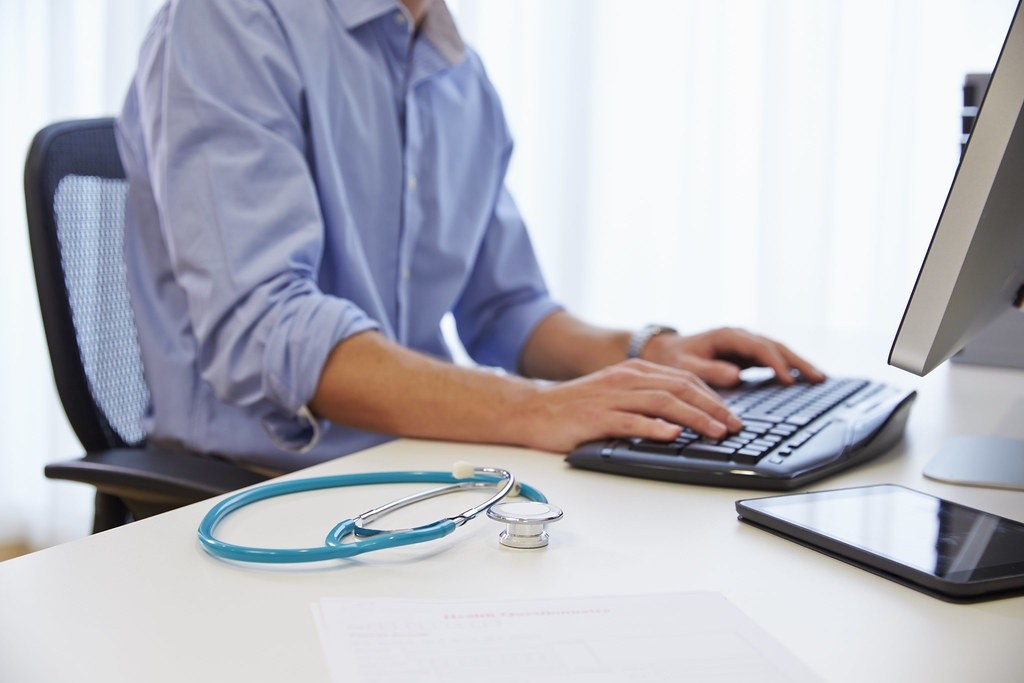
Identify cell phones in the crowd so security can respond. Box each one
[736,483,1024,597]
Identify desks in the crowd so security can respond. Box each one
[2,376,1022,681]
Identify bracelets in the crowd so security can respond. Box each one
[626,324,679,356]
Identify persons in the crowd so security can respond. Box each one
[113,0,827,525]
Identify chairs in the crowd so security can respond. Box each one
[23,116,275,537]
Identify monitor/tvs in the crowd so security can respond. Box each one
[888,0,1024,491]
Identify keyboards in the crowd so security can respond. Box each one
[564,369,917,493]
